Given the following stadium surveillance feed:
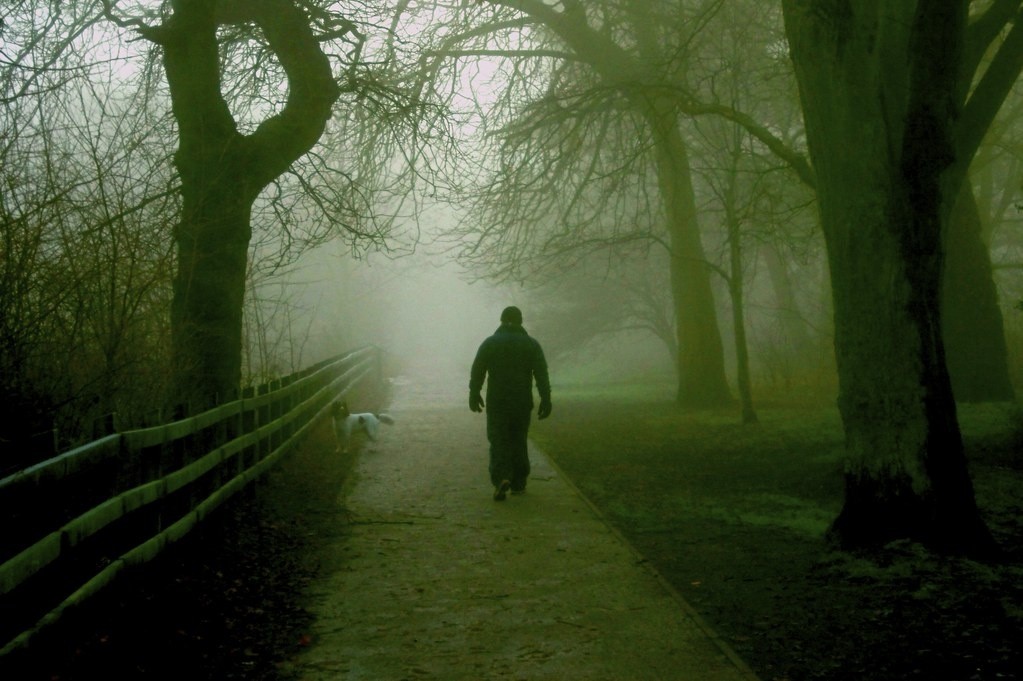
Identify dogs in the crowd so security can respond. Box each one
[328,400,395,454]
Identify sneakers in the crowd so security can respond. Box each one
[493,479,510,500]
[511,487,527,493]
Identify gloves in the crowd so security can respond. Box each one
[538,397,552,419]
[469,388,484,412]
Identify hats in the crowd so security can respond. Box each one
[500,305,522,323]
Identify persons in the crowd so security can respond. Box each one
[469,306,552,500]
